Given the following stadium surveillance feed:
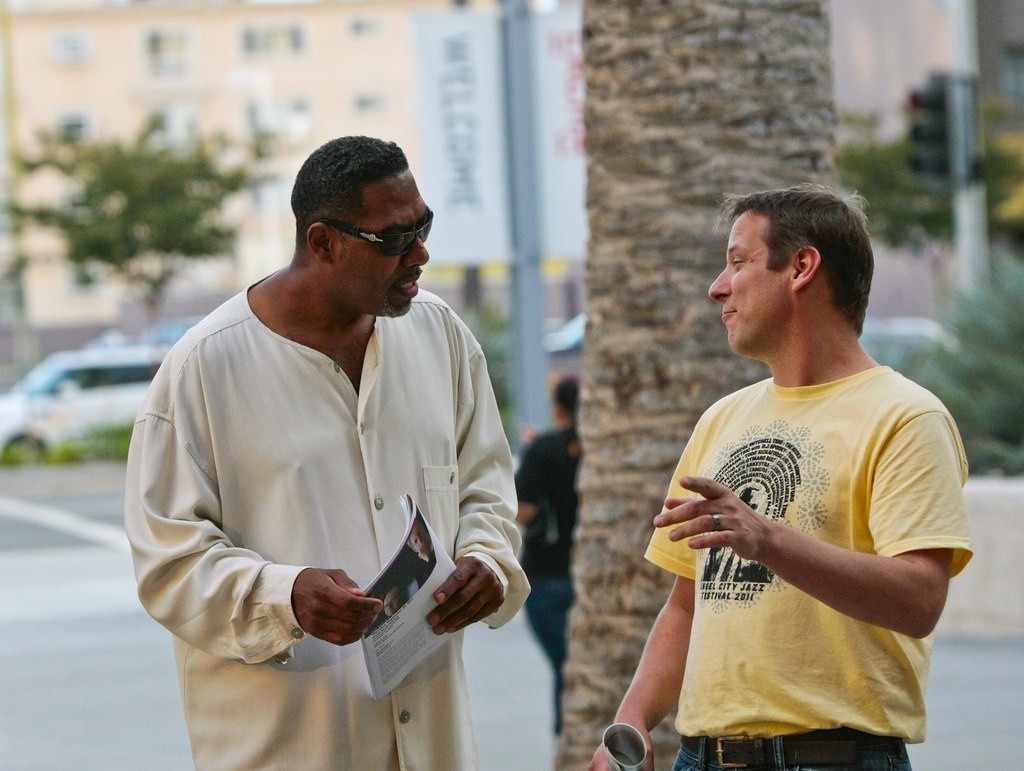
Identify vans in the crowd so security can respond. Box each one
[1,317,201,464]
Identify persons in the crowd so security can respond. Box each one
[384,588,407,615]
[513,375,582,732]
[584,181,977,771]
[407,520,436,588]
[122,136,534,771]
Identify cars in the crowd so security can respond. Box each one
[541,310,585,373]
[859,315,955,377]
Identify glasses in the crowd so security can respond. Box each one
[318,211,435,257]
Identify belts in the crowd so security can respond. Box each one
[681,729,897,769]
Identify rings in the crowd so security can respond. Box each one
[713,513,723,530]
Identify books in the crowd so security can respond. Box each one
[356,493,457,700]
